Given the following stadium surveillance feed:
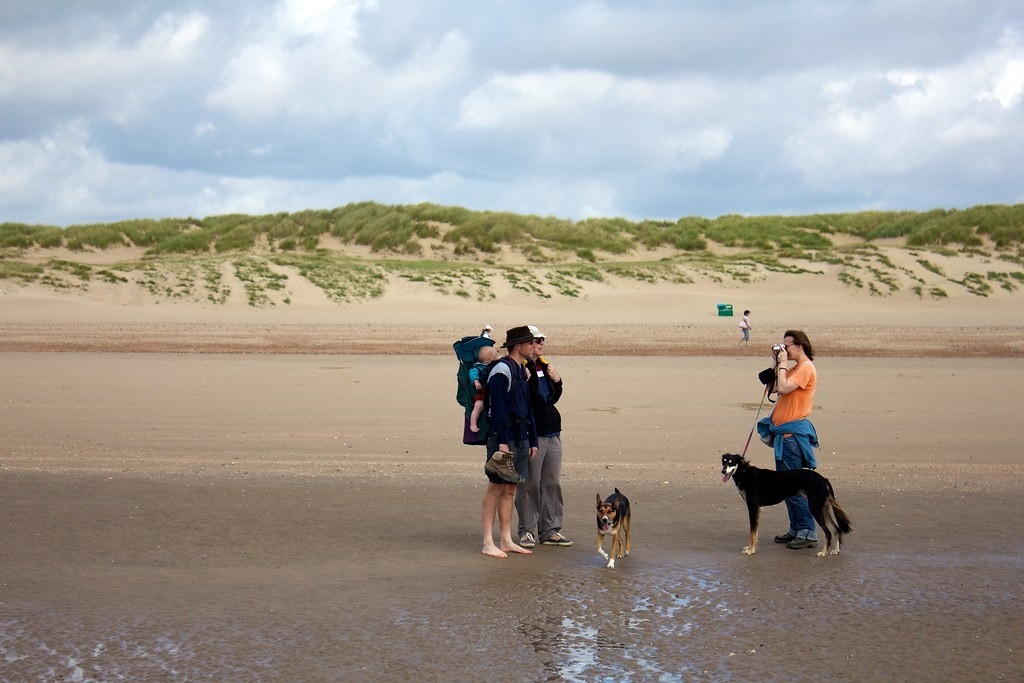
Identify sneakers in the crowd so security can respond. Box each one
[543,532,573,547]
[518,532,536,548]
[785,538,818,550]
[484,450,526,485]
[773,532,796,543]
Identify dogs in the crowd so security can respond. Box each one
[595,488,631,569]
[719,453,853,557]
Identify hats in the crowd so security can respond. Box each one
[484,325,492,331]
[499,325,535,348]
[527,325,547,340]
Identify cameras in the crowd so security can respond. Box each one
[772,343,785,353]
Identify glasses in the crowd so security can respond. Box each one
[533,338,544,344]
[524,340,533,345]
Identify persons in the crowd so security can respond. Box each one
[738,310,753,347]
[766,330,818,551]
[481,325,539,558]
[515,325,573,548]
[480,325,494,339]
[469,346,498,433]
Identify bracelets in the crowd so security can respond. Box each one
[779,368,787,372]
